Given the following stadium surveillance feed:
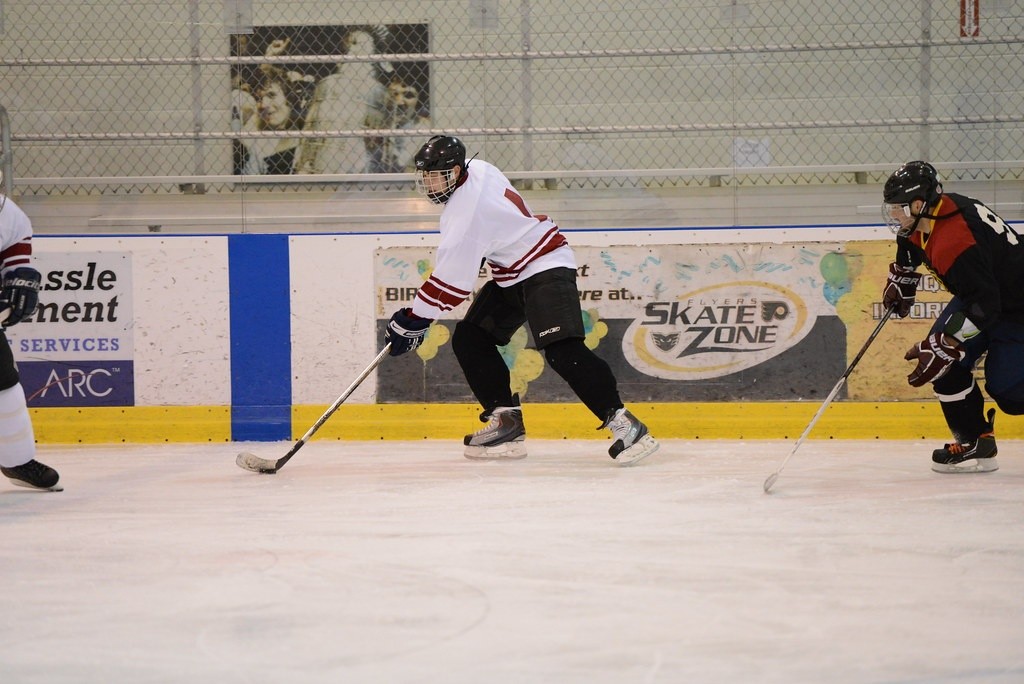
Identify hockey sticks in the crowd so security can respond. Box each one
[761,307,895,492]
[235,337,394,473]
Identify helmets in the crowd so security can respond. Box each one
[415,134,465,203]
[884,160,944,237]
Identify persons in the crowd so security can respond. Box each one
[883,160,1024,472]
[230,25,432,175]
[384,134,659,468]
[0,171,63,490]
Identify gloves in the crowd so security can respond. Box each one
[0,266,42,327]
[882,262,923,317]
[903,330,965,387]
[384,307,434,357]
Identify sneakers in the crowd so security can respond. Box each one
[931,408,999,473]
[464,391,528,461]
[1,461,63,492]
[596,404,660,465]
